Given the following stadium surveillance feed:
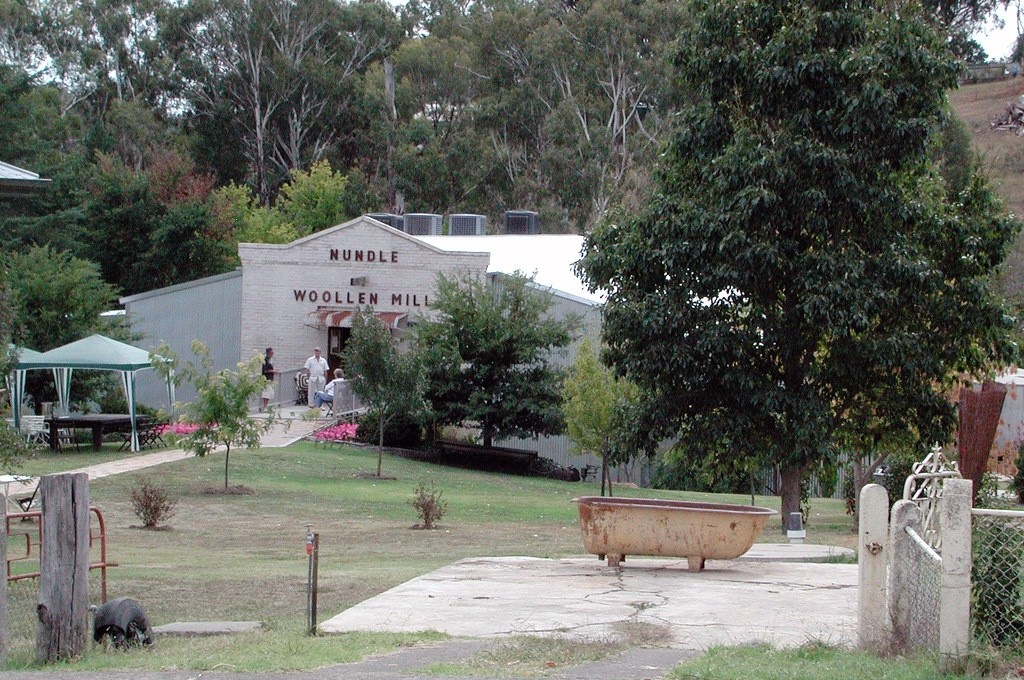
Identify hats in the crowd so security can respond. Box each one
[266,348,272,352]
[313,347,321,352]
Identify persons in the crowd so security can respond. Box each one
[260,347,279,412]
[304,348,330,410]
[313,369,345,411]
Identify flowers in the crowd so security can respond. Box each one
[69,402,81,413]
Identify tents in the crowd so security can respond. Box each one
[0,333,176,453]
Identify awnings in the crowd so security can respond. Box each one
[304,310,407,332]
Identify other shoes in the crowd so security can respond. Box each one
[320,406,325,411]
[264,407,271,413]
[309,407,313,410]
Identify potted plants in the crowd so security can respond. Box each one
[70,410,81,419]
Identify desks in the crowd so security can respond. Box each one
[0,474,32,515]
[44,414,148,453]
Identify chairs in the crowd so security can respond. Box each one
[294,370,309,406]
[324,400,333,418]
[15,476,42,521]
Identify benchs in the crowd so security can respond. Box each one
[117,418,169,452]
[6,414,79,453]
